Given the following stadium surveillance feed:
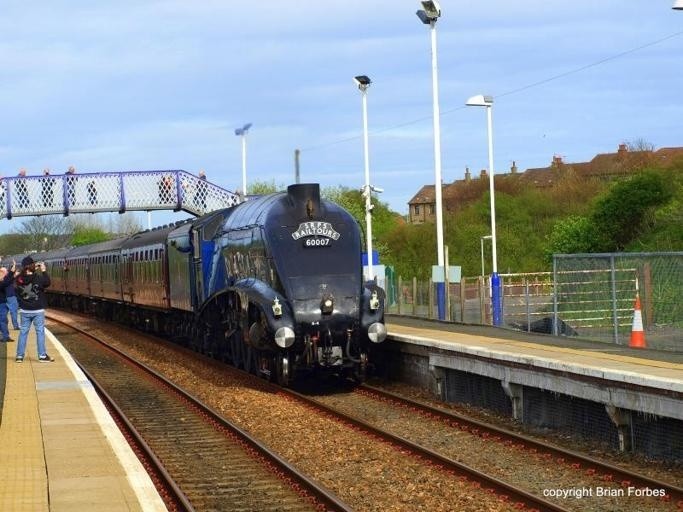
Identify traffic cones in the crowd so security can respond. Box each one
[628,292,646,347]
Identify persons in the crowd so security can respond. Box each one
[230,188,240,206]
[60,167,77,205]
[14,257,55,363]
[0,258,19,342]
[37,168,55,207]
[192,170,206,210]
[12,168,28,209]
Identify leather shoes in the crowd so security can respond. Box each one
[1,337,14,342]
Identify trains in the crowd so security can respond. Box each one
[0,183,387,389]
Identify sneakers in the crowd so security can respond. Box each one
[16,356,23,362]
[39,354,56,362]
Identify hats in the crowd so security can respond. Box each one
[22,257,37,266]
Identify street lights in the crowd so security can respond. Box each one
[466,95,501,329]
[234,122,252,202]
[416,1,444,320]
[353,74,384,281]
[671,1,683,11]
